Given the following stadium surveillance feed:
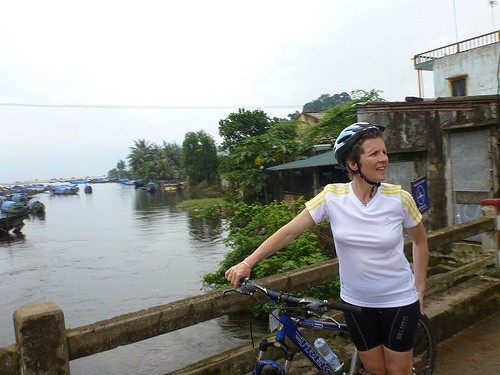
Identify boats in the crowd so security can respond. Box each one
[94,177,158,192]
[12,179,92,194]
[0,194,45,236]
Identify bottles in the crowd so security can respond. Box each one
[314,338,340,370]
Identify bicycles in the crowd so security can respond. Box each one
[221,269,435,375]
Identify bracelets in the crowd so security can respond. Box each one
[241,262,252,270]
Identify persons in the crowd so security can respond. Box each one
[225,122,428,375]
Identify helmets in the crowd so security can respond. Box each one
[334,122,386,167]
